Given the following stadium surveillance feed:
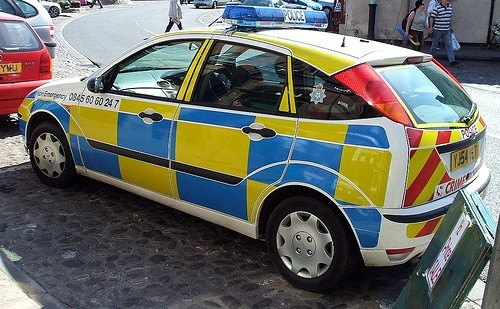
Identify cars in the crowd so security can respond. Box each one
[19,0,494,293]
[0,0,57,59]
[194,0,237,9]
[0,12,53,115]
[313,0,342,19]
[180,0,195,4]
[38,0,99,18]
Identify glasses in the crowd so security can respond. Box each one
[422,3,424,5]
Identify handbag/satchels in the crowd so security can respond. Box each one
[451,32,461,51]
[171,17,181,26]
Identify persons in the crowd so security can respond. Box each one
[427,0,459,66]
[165,0,183,33]
[89,0,103,9]
[405,0,431,51]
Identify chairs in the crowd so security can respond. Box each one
[230,66,263,89]
[197,68,229,102]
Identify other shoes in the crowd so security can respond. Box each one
[450,61,459,66]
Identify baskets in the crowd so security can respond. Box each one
[491,22,500,35]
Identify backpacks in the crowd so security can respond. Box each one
[402,9,416,33]
